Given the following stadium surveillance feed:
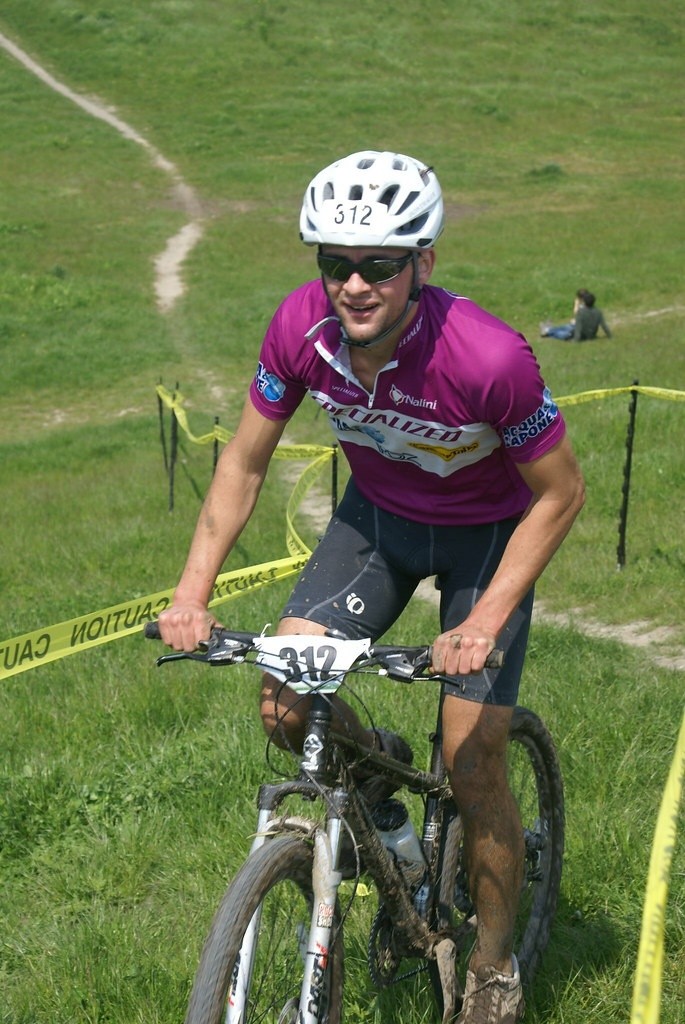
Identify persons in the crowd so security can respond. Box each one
[539,289,611,343]
[158,149,585,1024]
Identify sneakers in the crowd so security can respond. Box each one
[449,952,526,1024]
[336,729,414,880]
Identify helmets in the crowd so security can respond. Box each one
[299,150,444,252]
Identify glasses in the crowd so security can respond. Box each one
[318,252,421,285]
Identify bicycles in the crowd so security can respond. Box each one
[140,617,567,1023]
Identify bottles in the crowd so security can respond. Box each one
[369,796,429,890]
